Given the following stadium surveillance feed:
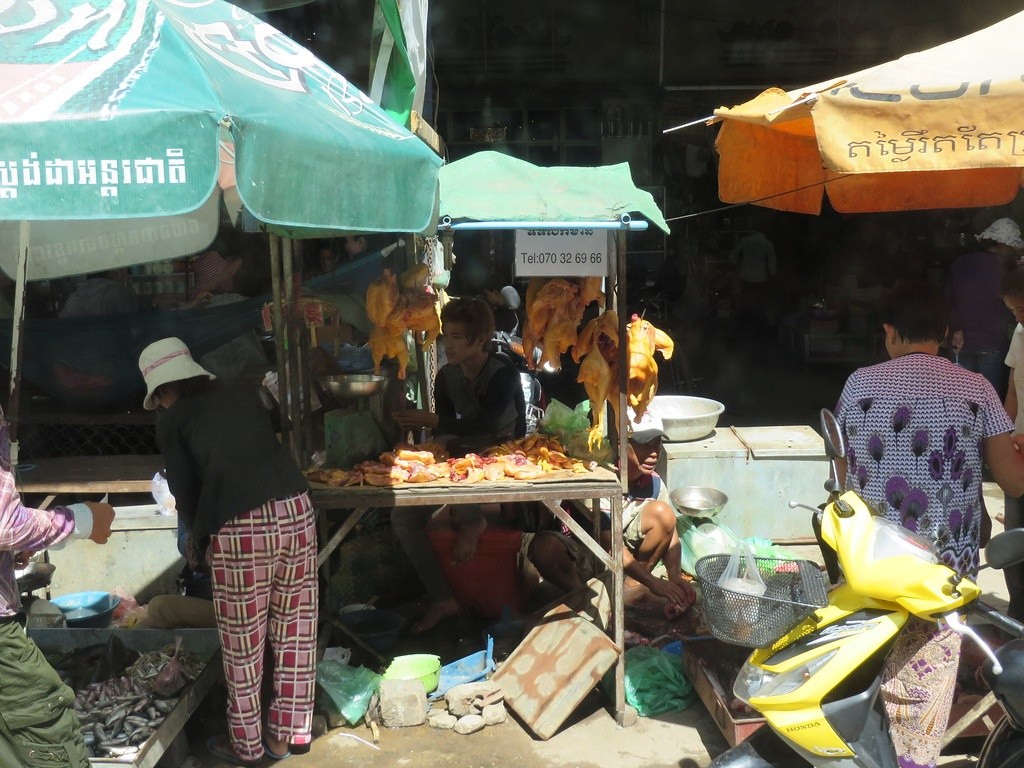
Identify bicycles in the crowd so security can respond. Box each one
[629,285,703,396]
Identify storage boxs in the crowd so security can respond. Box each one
[424,521,524,610]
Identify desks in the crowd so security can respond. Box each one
[303,470,623,708]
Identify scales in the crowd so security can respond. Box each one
[319,373,397,470]
[669,485,735,577]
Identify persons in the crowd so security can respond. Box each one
[833,283,1024,768]
[392,298,527,657]
[1002,253,1024,623]
[487,275,524,347]
[0,413,115,768]
[140,336,320,768]
[729,225,781,341]
[527,408,697,606]
[947,217,1024,397]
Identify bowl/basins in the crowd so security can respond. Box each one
[379,654,442,695]
[342,608,405,649]
[47,591,121,628]
[617,395,725,443]
[339,596,376,615]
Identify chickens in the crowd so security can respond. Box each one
[521,276,606,370]
[626,316,675,423]
[386,264,440,352]
[366,269,409,379]
[571,310,620,453]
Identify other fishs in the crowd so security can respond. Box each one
[72,642,206,760]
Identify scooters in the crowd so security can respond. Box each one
[489,283,565,442]
[694,408,1024,768]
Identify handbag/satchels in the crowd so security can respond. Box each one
[601,643,699,716]
[422,236,456,290]
[718,542,766,624]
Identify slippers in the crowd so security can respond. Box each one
[207,735,262,766]
[259,716,291,759]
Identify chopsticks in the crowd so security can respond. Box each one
[362,595,380,609]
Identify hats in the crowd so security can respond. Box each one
[976,218,1023,249]
[628,408,669,444]
[138,338,216,410]
[191,251,242,299]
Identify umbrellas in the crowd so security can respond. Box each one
[1,0,442,467]
[662,12,1023,211]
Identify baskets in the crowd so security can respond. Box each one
[696,553,829,648]
